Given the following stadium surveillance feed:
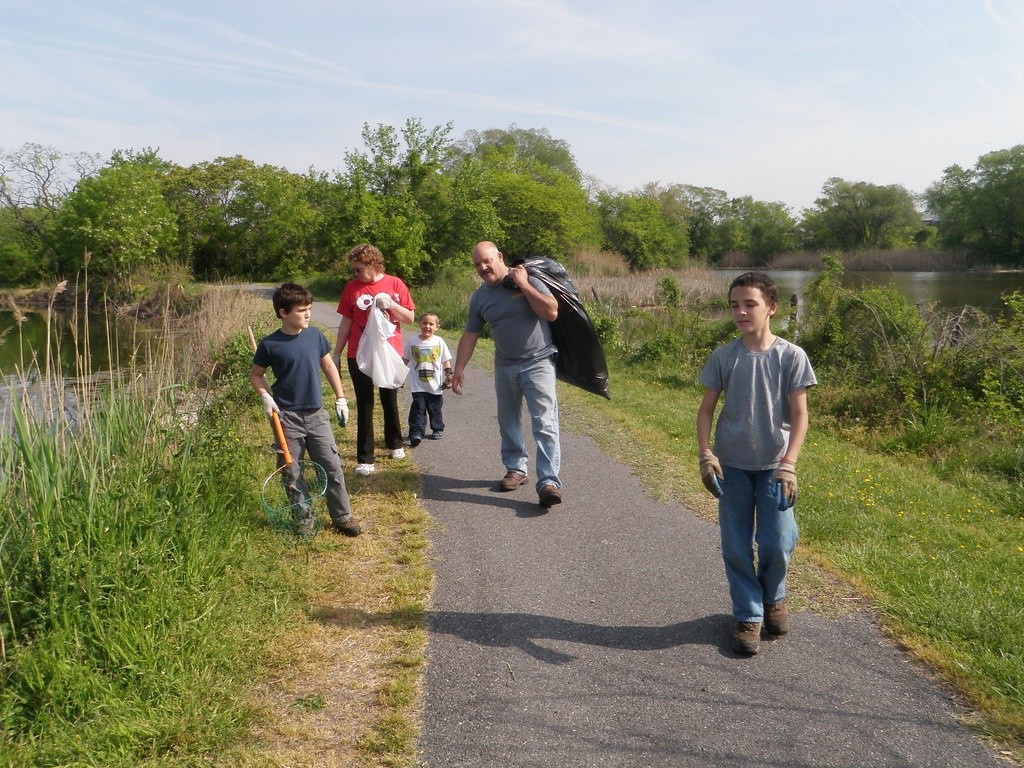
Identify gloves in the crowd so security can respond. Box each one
[332,354,342,379]
[698,448,724,498]
[373,292,392,310]
[335,396,349,428]
[771,457,798,511]
[261,393,284,422]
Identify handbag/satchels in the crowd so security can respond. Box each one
[355,298,411,389]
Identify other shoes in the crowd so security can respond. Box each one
[332,518,360,535]
[295,516,313,535]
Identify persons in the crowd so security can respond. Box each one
[696,271,818,654]
[250,284,361,537]
[402,311,453,446]
[452,241,562,506]
[332,244,415,477]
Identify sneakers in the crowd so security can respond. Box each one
[764,599,789,635]
[501,472,528,489]
[354,463,375,476]
[411,435,421,447]
[391,447,405,459]
[431,430,443,439]
[731,621,762,654]
[539,486,561,508]
